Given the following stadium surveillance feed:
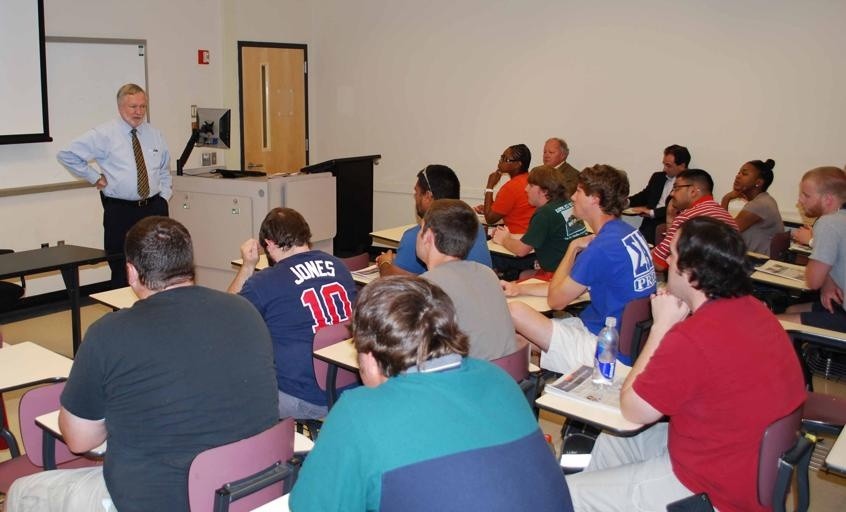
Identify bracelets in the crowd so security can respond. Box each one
[378,260,391,267]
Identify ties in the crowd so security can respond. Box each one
[130,128,150,199]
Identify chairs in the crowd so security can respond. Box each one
[0,249,26,304]
[187,416,296,512]
[667,400,817,512]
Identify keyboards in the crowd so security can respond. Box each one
[217,169,267,175]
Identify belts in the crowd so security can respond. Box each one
[137,199,148,207]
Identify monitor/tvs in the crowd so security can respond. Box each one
[177,108,231,175]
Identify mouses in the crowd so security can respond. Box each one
[210,169,217,173]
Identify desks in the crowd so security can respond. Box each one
[0,224,846,495]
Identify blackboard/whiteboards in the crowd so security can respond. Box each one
[0,35,151,197]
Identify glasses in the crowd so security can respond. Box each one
[420,167,432,193]
[501,155,518,162]
[673,184,692,191]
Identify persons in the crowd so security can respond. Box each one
[56,83,174,310]
[508,162,656,374]
[287,274,572,509]
[416,196,515,361]
[563,216,807,512]
[225,206,359,421]
[375,164,493,278]
[473,137,846,331]
[5,212,279,512]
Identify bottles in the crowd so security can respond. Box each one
[591,316,619,398]
